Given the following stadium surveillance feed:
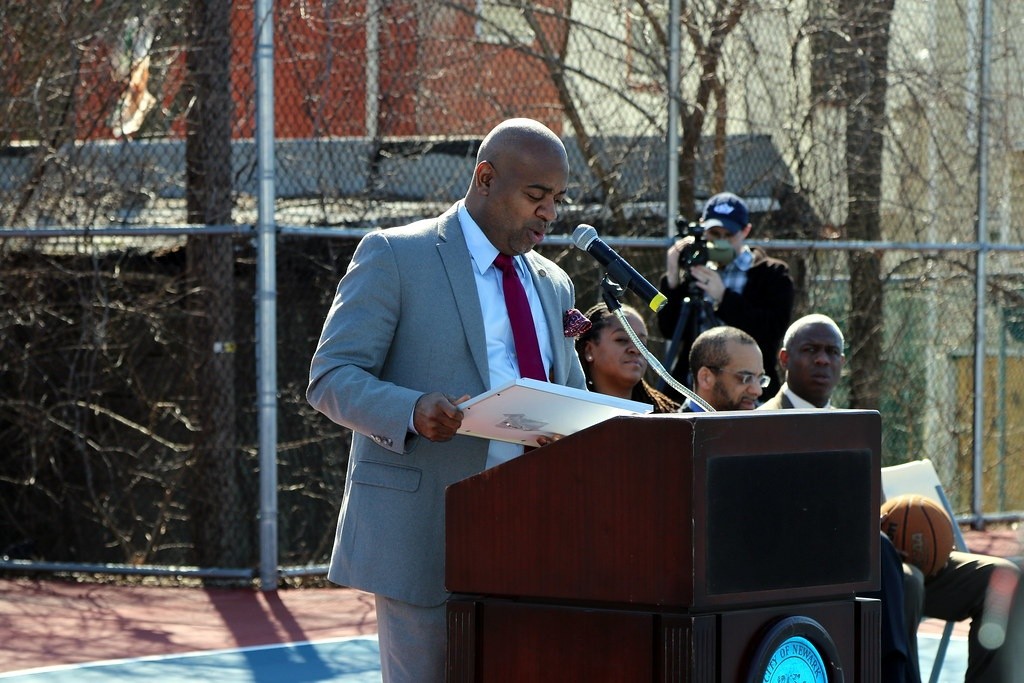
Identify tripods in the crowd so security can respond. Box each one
[653,272,724,414]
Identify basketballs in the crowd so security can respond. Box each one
[880,494,955,577]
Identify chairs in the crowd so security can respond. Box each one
[884,458,1024,683]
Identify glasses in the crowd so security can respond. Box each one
[708,366,771,388]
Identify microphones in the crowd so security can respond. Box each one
[572,224,668,313]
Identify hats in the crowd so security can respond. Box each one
[699,191,748,233]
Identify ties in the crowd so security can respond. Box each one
[492,253,547,383]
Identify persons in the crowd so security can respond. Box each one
[571,302,679,416]
[678,326,915,683]
[758,312,1024,683]
[303,113,593,683]
[654,191,794,401]
